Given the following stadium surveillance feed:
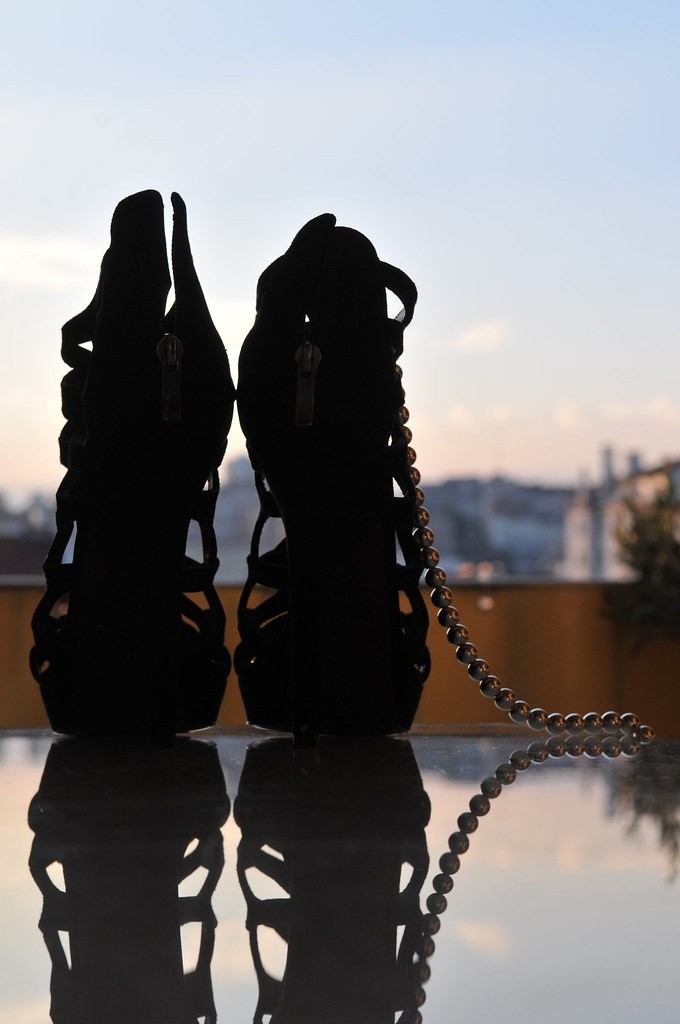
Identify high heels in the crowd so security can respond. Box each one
[26,189,432,735]
